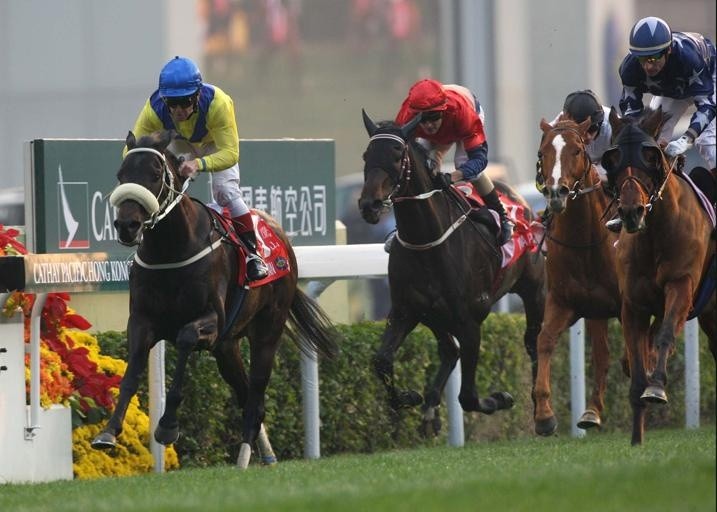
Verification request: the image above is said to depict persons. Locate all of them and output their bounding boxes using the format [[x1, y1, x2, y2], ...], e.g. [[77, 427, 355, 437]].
[[619, 15, 717, 179], [393, 78, 516, 247], [121, 54, 269, 282], [536, 88, 621, 203]]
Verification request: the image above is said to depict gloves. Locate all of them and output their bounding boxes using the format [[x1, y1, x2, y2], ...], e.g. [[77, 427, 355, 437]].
[[663, 135, 694, 160]]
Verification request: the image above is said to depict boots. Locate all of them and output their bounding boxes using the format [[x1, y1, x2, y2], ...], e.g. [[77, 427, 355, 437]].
[[240, 231, 269, 280], [480, 187, 517, 247]]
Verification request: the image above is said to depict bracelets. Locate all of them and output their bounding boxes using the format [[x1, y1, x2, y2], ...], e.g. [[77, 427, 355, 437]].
[[685, 131, 696, 144]]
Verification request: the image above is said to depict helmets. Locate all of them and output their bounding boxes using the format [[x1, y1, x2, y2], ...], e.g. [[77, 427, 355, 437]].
[[564, 89, 604, 127], [628, 17, 672, 56], [408, 80, 448, 114], [158, 56, 203, 97]]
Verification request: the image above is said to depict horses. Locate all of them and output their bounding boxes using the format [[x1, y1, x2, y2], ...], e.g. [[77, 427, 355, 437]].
[[533, 117, 676, 436], [600, 104, 717, 447], [90, 130, 339, 469], [358, 107, 551, 437]]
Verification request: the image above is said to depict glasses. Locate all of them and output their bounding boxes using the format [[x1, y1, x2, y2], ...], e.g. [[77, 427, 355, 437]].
[[160, 89, 200, 109], [416, 113, 442, 122], [635, 49, 666, 66]]
